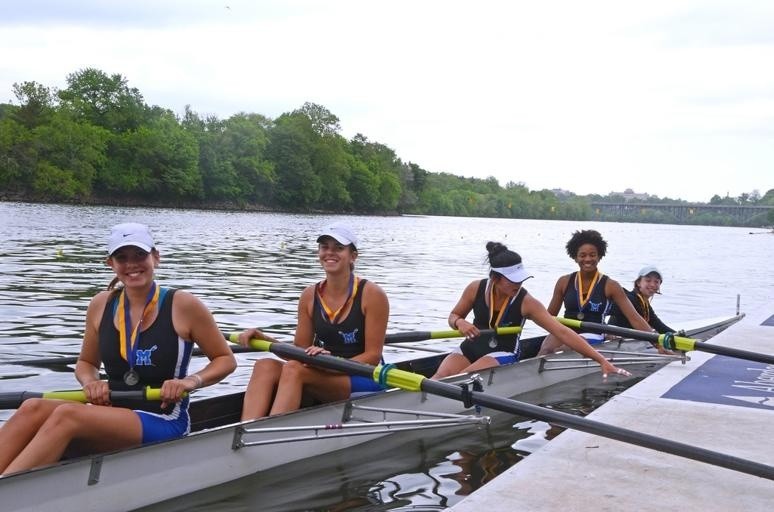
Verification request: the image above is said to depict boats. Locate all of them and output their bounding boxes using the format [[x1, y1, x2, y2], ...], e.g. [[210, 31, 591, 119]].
[[1, 294, 746, 510]]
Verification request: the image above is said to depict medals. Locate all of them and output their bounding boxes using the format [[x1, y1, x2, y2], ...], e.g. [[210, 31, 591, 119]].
[[575, 310, 585, 320], [123, 369, 141, 386], [488, 332, 503, 350]]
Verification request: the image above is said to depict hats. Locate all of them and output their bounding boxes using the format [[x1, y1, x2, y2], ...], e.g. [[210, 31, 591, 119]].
[[639, 267, 663, 283], [107, 223, 155, 256], [491, 262, 534, 283], [316, 226, 359, 251]]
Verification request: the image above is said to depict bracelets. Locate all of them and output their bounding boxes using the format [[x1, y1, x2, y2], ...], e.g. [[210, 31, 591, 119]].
[[186, 371, 209, 386]]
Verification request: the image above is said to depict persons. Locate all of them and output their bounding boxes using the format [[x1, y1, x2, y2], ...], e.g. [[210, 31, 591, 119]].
[[237, 224, 390, 426], [607, 264, 684, 340], [0, 222, 238, 482], [536, 227, 690, 365], [419, 242, 632, 385]]
